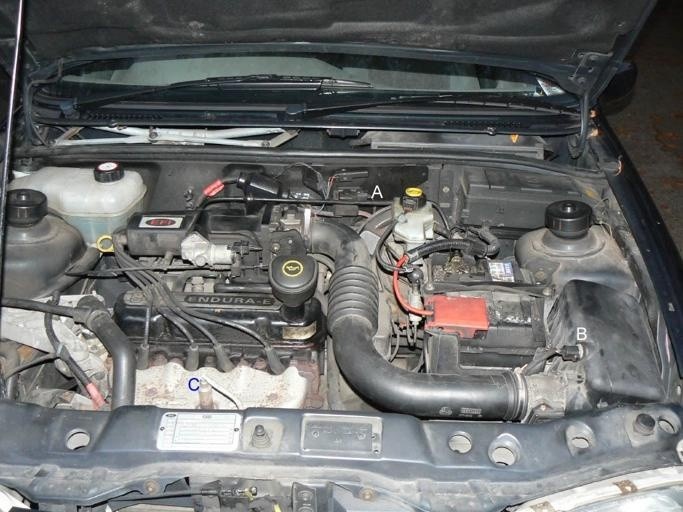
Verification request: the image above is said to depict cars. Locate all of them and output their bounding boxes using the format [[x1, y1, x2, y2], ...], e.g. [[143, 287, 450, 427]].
[[0, 0, 683, 512]]
